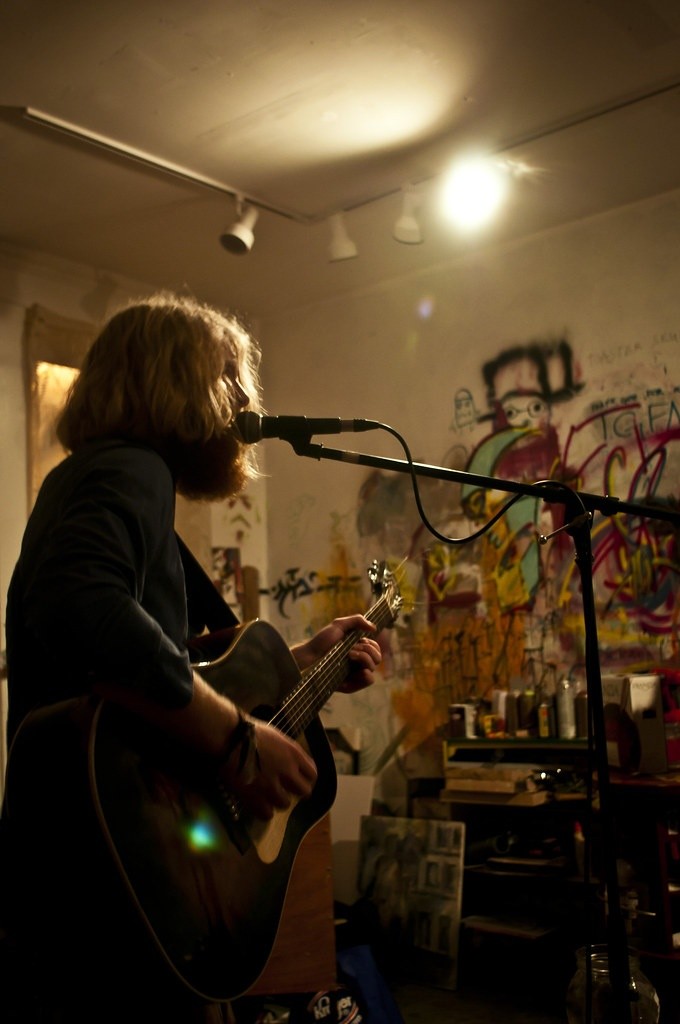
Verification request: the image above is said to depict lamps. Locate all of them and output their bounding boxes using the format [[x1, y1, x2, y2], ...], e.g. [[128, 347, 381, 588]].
[[392, 186, 422, 243], [329, 212, 359, 263], [221, 197, 260, 256]]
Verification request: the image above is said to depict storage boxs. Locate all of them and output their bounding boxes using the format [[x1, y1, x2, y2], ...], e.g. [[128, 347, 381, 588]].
[[442, 762, 547, 807], [447, 703, 477, 743]]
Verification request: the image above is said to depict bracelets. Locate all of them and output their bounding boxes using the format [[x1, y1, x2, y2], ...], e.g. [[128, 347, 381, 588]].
[[215, 707, 261, 778]]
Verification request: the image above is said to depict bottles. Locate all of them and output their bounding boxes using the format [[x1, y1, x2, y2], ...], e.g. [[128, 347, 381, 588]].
[[555, 680, 576, 739]]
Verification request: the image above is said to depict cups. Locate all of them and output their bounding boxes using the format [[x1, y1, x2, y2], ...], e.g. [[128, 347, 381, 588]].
[[448, 703, 475, 737]]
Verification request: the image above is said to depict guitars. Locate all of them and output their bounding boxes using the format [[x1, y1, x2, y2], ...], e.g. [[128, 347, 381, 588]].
[[0, 556, 402, 1014]]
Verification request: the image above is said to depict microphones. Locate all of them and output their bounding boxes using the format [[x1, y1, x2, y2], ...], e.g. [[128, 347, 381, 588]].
[[231, 410, 382, 445]]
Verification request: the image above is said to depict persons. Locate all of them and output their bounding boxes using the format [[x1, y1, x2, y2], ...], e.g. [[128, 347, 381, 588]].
[[0, 303, 383, 1024]]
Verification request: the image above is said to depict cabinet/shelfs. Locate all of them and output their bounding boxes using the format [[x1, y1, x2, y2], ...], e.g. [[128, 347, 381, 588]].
[[596, 772, 680, 968], [446, 736, 616, 998]]
